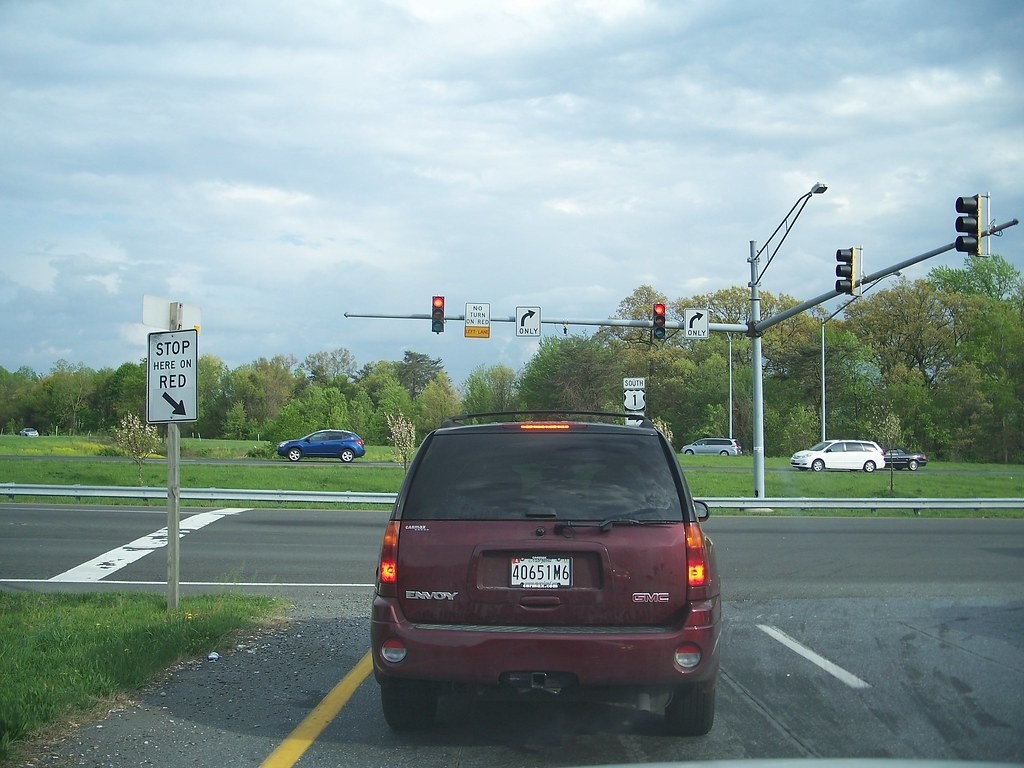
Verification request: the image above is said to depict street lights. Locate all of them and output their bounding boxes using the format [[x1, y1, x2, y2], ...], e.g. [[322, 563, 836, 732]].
[[747, 181, 829, 497], [821, 270, 902, 443]]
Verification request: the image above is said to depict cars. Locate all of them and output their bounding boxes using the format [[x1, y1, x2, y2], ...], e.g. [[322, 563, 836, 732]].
[[680, 438, 742, 458], [277, 429, 366, 463], [19, 427, 40, 439], [882, 448, 929, 471]]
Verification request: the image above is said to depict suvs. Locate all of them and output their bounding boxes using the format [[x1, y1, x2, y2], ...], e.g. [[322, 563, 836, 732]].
[[369, 409, 721, 735], [789, 439, 887, 472]]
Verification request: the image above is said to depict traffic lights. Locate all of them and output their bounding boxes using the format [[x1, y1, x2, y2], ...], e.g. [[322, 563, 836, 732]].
[[431, 296, 445, 333], [835, 247, 856, 295], [955, 193, 983, 258], [652, 301, 668, 341]]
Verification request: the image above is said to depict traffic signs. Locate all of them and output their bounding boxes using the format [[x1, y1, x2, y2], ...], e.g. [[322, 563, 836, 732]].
[[147, 329, 199, 425]]
